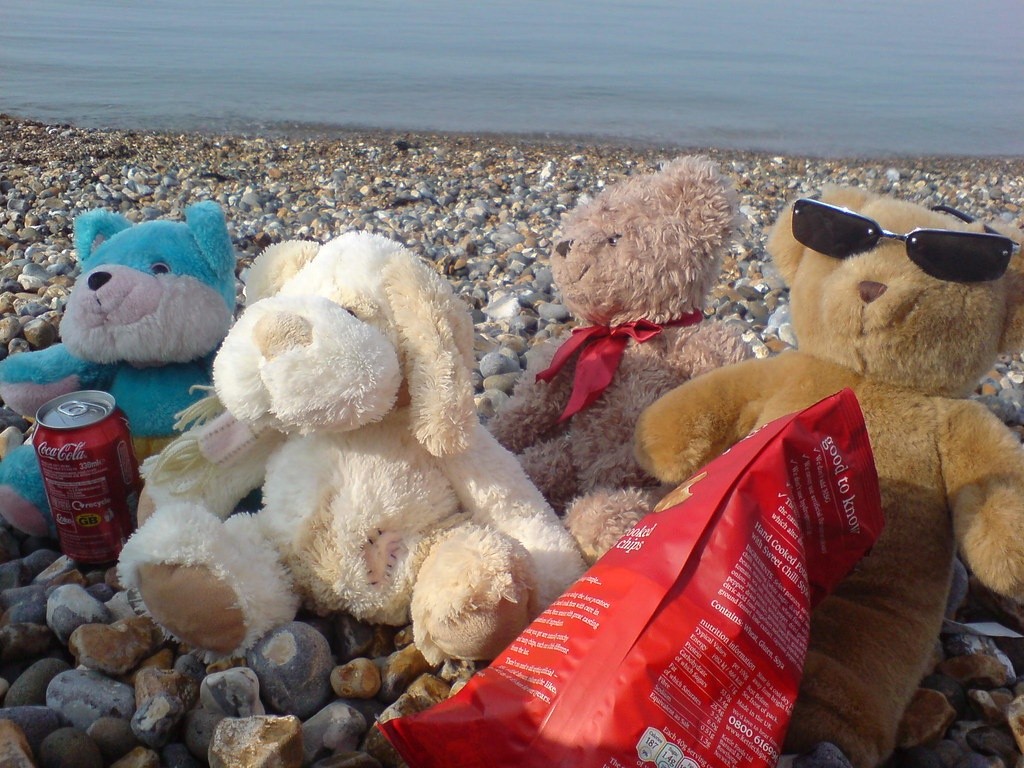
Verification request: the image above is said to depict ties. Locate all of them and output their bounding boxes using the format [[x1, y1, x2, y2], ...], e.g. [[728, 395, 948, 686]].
[[526, 314, 713, 416]]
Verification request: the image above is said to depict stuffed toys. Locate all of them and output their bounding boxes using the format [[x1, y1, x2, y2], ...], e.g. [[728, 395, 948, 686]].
[[0, 200, 261, 537], [484, 155, 755, 569], [117, 232, 590, 678], [634, 188, 1024, 768]]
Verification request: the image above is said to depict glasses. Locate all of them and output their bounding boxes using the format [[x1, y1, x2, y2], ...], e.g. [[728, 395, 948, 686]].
[[792, 191, 1018, 285]]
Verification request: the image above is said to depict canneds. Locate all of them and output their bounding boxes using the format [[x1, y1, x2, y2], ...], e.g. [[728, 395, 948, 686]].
[[35, 389, 143, 567]]
